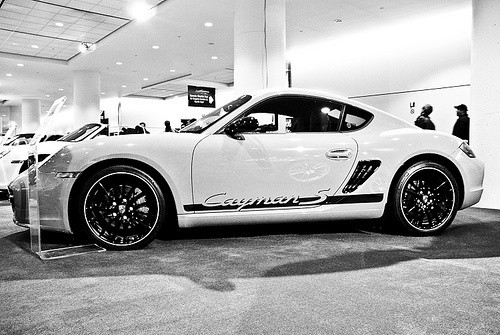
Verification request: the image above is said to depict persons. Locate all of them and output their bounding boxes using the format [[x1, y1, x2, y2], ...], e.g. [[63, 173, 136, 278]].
[[137, 118, 197, 134], [451, 103, 470, 145], [414, 104, 436, 130]]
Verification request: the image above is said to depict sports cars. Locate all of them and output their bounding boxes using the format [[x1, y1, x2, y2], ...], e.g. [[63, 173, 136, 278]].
[[0, 121, 109, 191], [6, 88, 486, 251]]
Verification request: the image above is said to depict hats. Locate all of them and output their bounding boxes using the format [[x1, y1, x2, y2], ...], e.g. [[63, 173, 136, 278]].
[[454, 104, 467, 112]]
